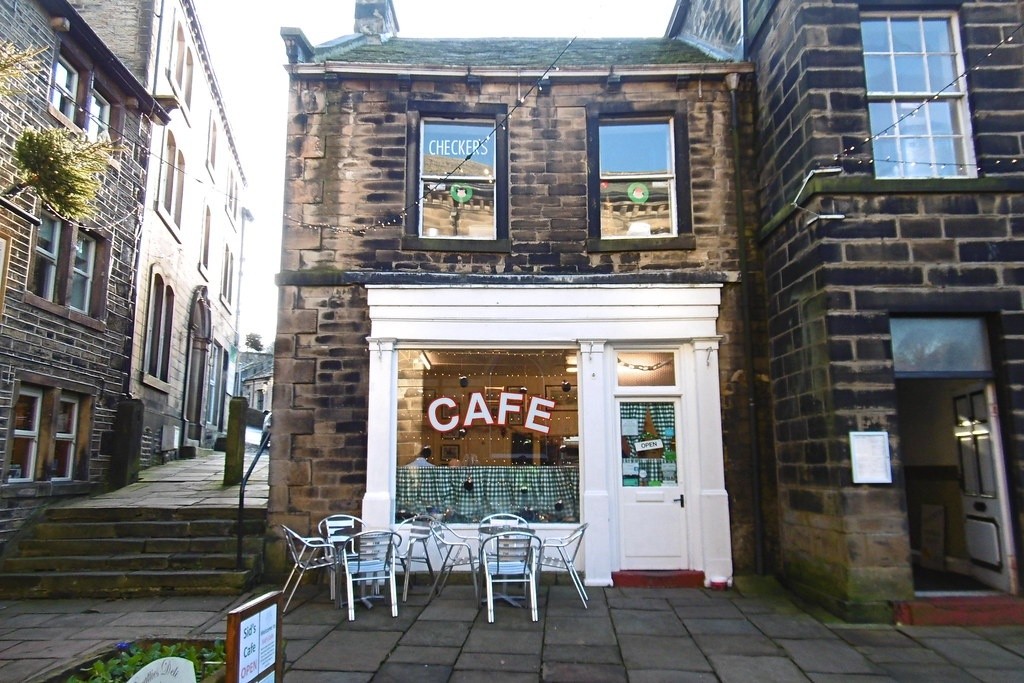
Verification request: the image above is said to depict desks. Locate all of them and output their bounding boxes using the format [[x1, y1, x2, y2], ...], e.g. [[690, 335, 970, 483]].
[[478, 526, 536, 608], [332, 528, 386, 601]]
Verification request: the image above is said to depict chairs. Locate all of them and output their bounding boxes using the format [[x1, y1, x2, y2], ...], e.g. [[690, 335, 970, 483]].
[[535, 522, 590, 609], [480, 532, 543, 623], [318, 514, 370, 608], [344, 529, 403, 621], [280, 525, 340, 613], [477, 512, 532, 606], [428, 520, 480, 604], [388, 515, 440, 604]]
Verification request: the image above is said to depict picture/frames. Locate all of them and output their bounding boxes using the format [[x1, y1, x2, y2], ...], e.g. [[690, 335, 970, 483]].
[[441, 402, 461, 420], [508, 407, 524, 426], [441, 443, 460, 461], [424, 388, 436, 399], [483, 385, 504, 402], [441, 427, 461, 439], [507, 386, 525, 405], [544, 384, 578, 412]]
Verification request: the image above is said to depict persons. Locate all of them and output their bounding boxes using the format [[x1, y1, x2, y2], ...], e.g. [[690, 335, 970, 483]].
[[447, 450, 461, 466], [260, 410, 272, 448], [408, 448, 436, 466]]
